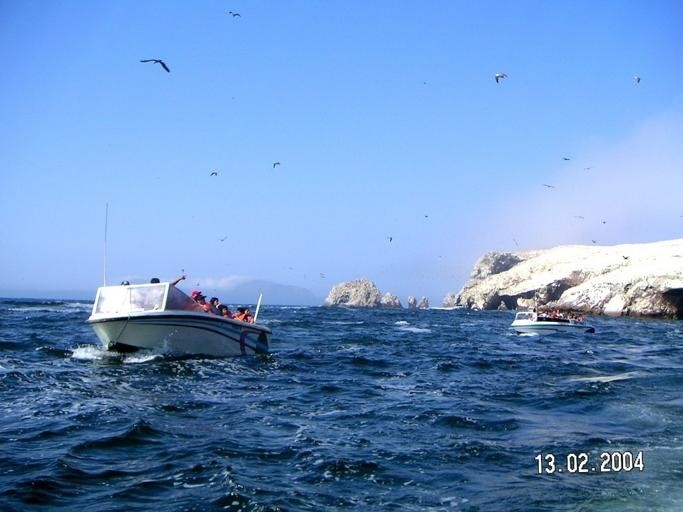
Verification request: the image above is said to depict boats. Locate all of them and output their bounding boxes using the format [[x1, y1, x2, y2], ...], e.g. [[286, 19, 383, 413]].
[[511, 311, 594, 336], [85, 283, 272, 356]]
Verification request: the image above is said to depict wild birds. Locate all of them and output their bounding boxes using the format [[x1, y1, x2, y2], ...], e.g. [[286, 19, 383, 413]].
[[227, 10, 239, 17], [138, 58, 169, 73], [272, 161, 280, 168], [208, 170, 217, 178], [495, 73, 507, 84], [634, 76, 641, 85]]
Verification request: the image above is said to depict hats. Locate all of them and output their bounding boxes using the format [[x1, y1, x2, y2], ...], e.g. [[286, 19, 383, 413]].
[[196, 296, 207, 301], [192, 290, 201, 296]]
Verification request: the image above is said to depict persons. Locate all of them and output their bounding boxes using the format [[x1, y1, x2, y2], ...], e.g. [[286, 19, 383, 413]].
[[121, 276, 254, 323], [540, 303, 584, 321]]
